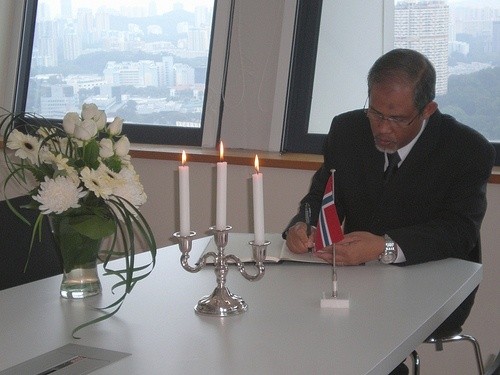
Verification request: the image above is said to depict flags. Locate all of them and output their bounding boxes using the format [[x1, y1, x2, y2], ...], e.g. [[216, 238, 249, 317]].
[[315, 176, 344, 251]]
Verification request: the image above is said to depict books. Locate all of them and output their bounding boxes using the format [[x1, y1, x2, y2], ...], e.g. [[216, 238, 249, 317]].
[[195, 233, 329, 265]]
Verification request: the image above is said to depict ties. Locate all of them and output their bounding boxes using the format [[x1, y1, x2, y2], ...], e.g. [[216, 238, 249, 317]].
[[383, 150, 401, 191]]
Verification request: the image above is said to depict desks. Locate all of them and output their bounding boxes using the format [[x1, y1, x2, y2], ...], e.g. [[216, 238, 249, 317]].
[[0, 233, 483, 375]]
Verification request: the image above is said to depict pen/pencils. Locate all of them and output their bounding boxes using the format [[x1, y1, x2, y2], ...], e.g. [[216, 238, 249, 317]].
[[305, 203, 312, 255], [331, 242, 338, 297]]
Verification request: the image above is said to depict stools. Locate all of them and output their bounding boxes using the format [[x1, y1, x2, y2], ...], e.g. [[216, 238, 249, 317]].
[[409, 326, 486, 375]]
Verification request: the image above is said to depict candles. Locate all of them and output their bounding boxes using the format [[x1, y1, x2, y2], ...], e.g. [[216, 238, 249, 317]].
[[216, 140, 227, 229], [251, 153, 264, 243], [178, 149, 191, 236]]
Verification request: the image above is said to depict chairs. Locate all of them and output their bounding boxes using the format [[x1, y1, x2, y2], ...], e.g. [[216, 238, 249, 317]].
[[0, 194, 63, 290]]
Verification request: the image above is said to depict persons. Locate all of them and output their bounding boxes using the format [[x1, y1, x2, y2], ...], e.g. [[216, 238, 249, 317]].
[[282, 48, 494, 375]]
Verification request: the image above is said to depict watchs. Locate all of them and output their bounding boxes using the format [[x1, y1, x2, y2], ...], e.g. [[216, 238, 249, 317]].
[[377, 233, 396, 265]]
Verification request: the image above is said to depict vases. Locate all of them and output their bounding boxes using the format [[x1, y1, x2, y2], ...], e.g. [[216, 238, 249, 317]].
[[45, 212, 101, 299]]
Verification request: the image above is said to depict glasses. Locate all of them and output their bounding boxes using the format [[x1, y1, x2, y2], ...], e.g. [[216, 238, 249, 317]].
[[363, 96, 426, 126]]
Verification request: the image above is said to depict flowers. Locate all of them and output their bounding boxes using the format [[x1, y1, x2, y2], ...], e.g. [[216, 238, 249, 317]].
[[0, 103, 156, 340]]
